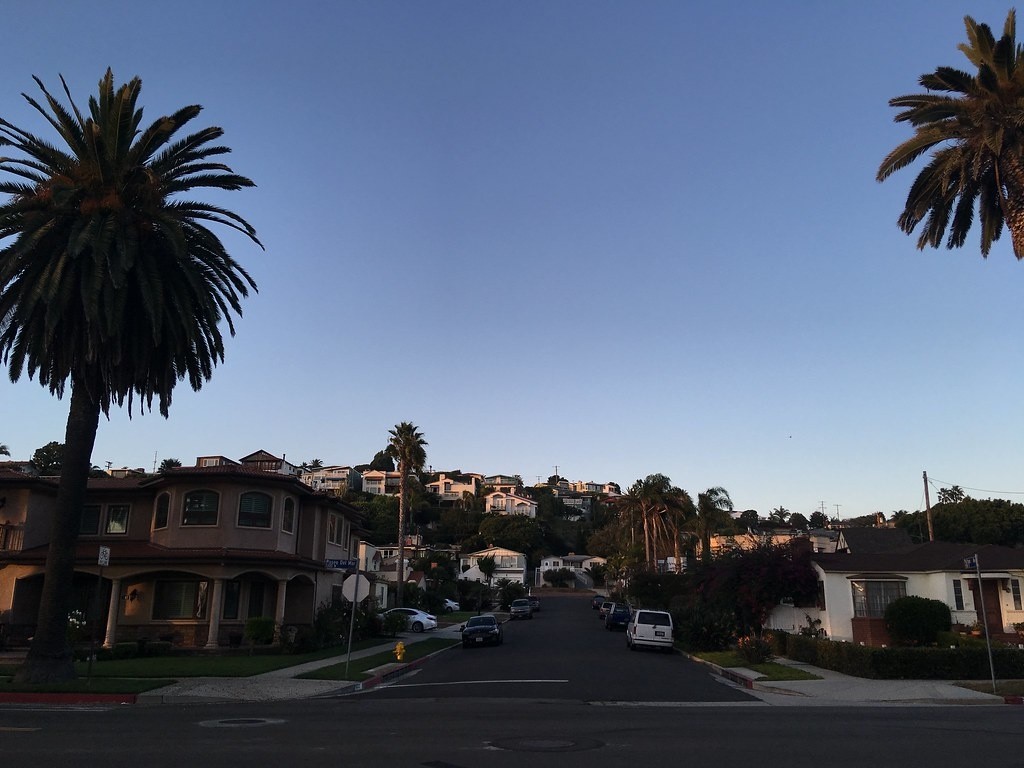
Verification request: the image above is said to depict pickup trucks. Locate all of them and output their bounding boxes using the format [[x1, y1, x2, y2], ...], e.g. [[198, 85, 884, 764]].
[[605, 603, 631, 631]]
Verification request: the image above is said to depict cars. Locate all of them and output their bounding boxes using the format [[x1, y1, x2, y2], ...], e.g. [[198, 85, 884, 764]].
[[600, 602, 615, 619], [508, 599, 532, 620], [442, 599, 460, 612], [592, 595, 606, 610], [376, 607, 437, 633], [530, 601, 541, 611], [460, 615, 504, 646]]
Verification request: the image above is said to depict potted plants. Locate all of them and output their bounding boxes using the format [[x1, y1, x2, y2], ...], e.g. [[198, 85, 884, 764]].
[[971, 620, 985, 638], [1013, 622, 1024, 639], [952, 615, 965, 632]]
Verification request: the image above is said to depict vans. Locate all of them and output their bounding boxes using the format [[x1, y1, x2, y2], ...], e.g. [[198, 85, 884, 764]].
[[626, 609, 674, 652]]
